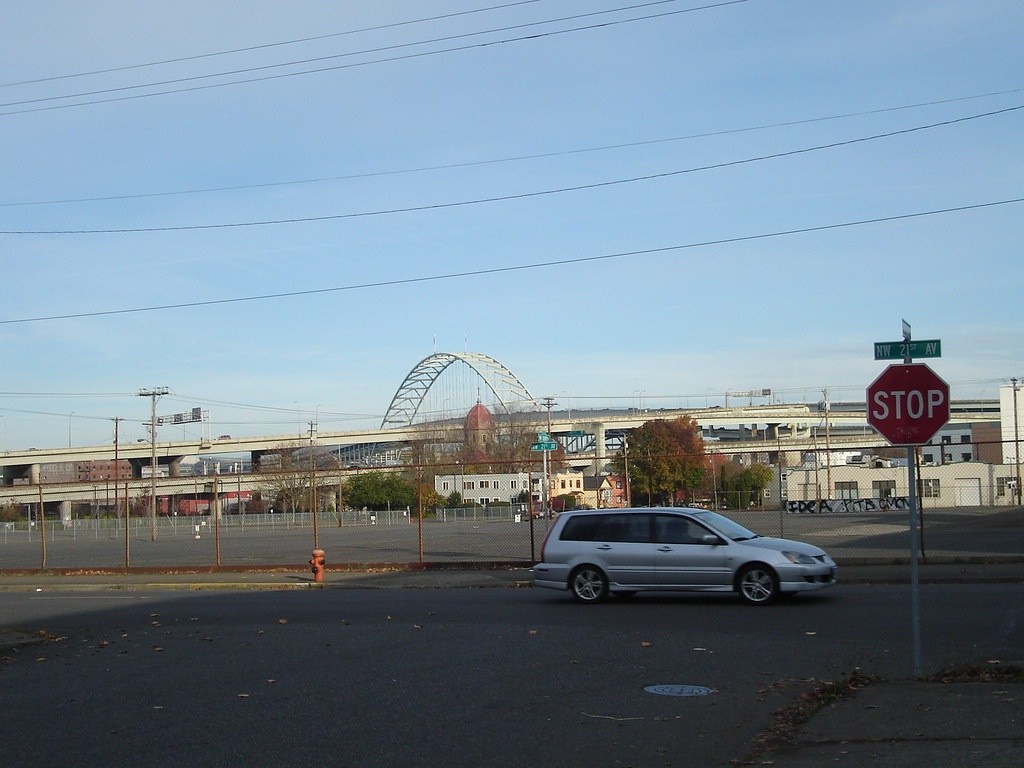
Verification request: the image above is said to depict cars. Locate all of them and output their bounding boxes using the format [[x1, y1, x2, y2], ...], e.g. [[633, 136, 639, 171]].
[[532, 505, 841, 606]]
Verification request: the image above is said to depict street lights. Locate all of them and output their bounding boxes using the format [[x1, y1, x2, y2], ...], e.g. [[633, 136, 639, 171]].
[[725, 386, 734, 408], [632, 390, 640, 413], [68, 411, 75, 449], [639, 389, 647, 411], [135, 437, 157, 542], [706, 387, 714, 408], [316, 403, 322, 433], [443, 398, 450, 419]]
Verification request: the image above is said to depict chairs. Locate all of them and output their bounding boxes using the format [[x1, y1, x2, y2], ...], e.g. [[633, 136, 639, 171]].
[[665, 519, 686, 545]]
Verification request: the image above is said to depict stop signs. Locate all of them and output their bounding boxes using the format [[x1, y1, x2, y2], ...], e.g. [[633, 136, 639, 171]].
[[864, 364, 952, 446]]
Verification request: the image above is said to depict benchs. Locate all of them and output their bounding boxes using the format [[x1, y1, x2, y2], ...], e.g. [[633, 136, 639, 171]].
[[569, 522, 636, 543]]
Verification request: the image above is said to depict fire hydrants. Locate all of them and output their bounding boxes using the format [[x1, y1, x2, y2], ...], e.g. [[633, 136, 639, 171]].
[[308, 549, 325, 583]]
